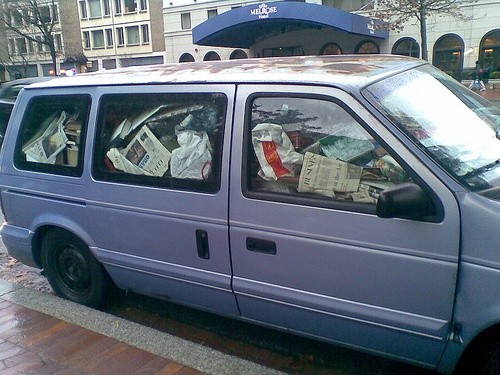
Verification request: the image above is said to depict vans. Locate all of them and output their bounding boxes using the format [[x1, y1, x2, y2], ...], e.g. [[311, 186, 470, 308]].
[[0, 54, 500, 374]]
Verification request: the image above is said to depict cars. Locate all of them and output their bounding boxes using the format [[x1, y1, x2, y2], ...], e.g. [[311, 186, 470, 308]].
[[0, 77, 59, 146]]
